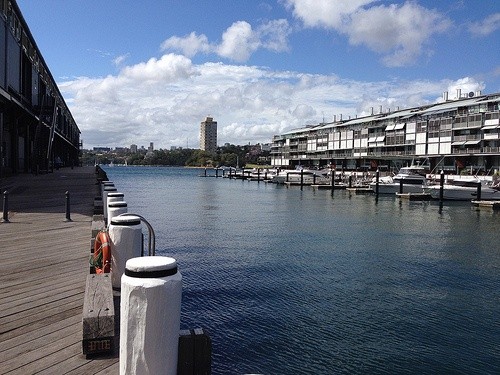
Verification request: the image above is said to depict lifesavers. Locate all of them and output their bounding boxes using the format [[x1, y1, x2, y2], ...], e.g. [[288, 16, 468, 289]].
[[93, 230, 111, 274]]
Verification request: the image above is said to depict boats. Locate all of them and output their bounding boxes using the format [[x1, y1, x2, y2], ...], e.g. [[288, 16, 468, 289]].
[[215, 153, 500, 200]]
